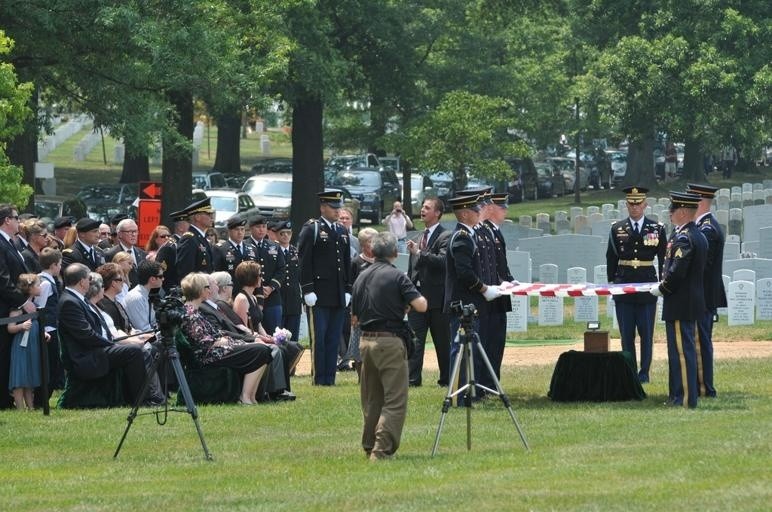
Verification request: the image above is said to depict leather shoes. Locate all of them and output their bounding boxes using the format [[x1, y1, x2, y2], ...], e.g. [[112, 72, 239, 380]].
[[276, 392, 289, 400], [637, 369, 649, 382], [283, 388, 297, 401]]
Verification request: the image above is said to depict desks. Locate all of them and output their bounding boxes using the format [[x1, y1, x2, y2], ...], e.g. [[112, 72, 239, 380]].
[[545, 349, 646, 401]]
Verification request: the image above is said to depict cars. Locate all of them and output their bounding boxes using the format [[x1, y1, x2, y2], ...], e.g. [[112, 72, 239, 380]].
[[205, 187, 259, 231], [74, 183, 135, 224], [193, 170, 230, 195], [33, 193, 89, 221]]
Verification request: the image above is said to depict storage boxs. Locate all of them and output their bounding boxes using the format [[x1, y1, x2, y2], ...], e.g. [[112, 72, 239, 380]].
[[584, 331, 610, 352]]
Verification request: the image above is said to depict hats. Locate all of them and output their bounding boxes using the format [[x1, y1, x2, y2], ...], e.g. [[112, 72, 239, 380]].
[[52, 215, 77, 227], [76, 216, 103, 233], [685, 183, 719, 200], [667, 192, 703, 209], [111, 213, 128, 225], [316, 190, 345, 210], [19, 212, 38, 222], [621, 185, 647, 203], [448, 185, 509, 210], [225, 215, 294, 232], [169, 197, 216, 221]]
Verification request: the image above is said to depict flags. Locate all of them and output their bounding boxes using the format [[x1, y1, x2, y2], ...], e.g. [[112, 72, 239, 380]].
[[499, 281, 661, 297]]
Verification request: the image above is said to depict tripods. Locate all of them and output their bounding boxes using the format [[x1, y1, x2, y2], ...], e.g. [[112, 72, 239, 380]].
[[114, 338, 214, 463], [432, 323, 533, 462]]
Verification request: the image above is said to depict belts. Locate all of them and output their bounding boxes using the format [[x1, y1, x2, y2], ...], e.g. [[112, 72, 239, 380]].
[[361, 330, 398, 337]]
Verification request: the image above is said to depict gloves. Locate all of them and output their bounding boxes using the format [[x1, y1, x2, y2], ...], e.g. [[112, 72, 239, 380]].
[[650, 281, 660, 295], [486, 281, 516, 300], [304, 292, 317, 306]]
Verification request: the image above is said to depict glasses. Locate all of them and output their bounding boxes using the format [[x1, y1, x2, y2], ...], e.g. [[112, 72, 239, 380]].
[[150, 274, 163, 279], [2, 215, 18, 220], [39, 233, 47, 238]]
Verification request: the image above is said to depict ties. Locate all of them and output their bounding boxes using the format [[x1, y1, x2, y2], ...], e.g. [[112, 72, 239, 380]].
[[127, 249, 131, 255], [257, 242, 261, 248], [634, 222, 639, 233], [421, 229, 430, 250], [89, 248, 93, 258], [284, 248, 288, 255], [330, 224, 335, 233], [236, 245, 242, 254]]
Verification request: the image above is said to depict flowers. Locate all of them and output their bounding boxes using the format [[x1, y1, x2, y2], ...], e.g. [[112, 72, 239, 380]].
[[270, 325, 293, 350]]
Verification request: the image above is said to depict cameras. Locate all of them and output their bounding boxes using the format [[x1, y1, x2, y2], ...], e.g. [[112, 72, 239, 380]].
[[452, 301, 479, 323]]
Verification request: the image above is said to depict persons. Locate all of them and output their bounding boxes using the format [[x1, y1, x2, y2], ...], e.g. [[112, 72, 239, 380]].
[[650, 189, 709, 409], [606, 185, 668, 382], [684, 184, 728, 397]]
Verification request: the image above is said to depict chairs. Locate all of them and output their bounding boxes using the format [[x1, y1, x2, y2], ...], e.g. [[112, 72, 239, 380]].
[[55, 320, 263, 410]]
